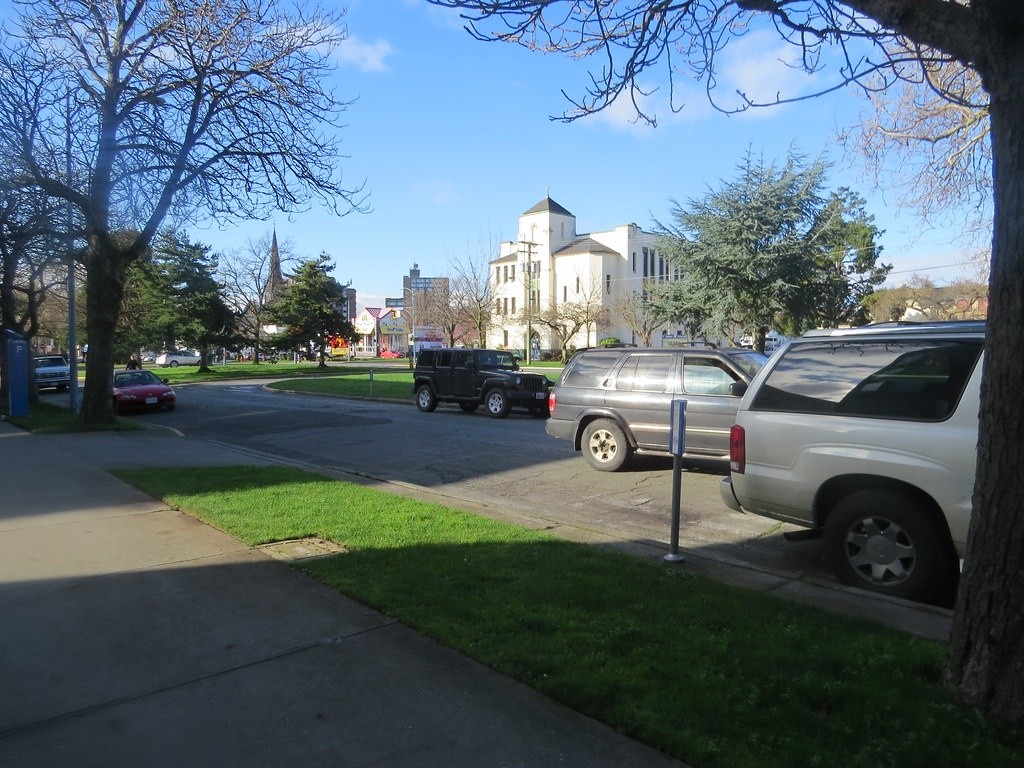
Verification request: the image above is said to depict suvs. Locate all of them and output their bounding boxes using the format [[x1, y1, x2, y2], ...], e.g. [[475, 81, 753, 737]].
[[718, 316, 988, 604], [410, 346, 552, 420], [32, 355, 71, 390], [542, 341, 775, 472]]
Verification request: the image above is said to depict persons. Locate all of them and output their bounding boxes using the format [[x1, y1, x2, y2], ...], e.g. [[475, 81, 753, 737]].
[[126, 356, 140, 370]]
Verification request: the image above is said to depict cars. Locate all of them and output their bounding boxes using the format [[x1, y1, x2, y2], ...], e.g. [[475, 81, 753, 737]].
[[112, 368, 176, 414], [139, 353, 156, 363], [242, 352, 279, 365], [157, 348, 202, 368], [381, 350, 404, 359]]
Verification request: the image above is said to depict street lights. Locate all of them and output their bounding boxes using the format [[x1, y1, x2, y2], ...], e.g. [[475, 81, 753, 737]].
[[62, 85, 167, 415], [399, 286, 417, 368]]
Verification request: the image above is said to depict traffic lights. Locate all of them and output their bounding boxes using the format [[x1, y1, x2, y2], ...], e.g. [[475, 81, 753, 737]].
[[391, 308, 397, 319]]
